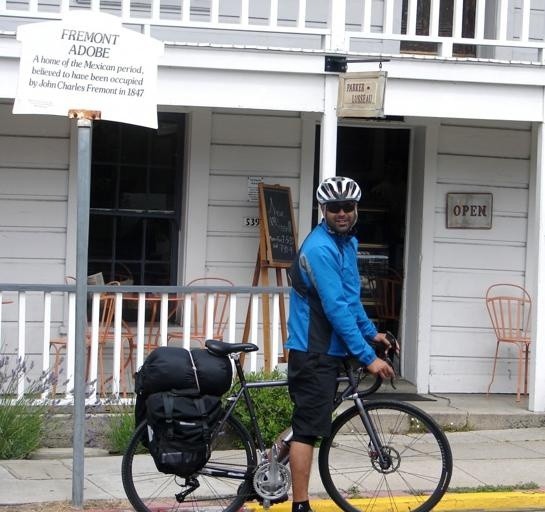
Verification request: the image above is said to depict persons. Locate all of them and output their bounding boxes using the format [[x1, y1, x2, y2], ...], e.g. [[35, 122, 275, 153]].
[[258, 175, 402, 512]]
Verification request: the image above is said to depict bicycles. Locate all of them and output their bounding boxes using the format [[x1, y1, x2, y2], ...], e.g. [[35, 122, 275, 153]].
[[122, 335, 454, 512]]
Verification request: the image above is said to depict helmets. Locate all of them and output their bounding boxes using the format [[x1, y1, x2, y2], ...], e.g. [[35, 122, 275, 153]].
[[315, 175, 363, 205]]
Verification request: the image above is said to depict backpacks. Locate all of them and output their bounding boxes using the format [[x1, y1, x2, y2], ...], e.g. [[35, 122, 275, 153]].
[[145, 391, 223, 481]]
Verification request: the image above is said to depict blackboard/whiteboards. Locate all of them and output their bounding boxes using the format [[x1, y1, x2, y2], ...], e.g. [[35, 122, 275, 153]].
[[258, 184, 299, 268]]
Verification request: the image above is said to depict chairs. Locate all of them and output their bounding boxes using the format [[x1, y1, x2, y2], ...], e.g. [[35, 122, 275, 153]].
[[485, 283, 532, 406], [51, 276, 236, 403], [363, 268, 402, 372]]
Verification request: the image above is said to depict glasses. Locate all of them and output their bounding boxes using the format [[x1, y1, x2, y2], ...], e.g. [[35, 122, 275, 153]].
[[327, 203, 354, 214]]
